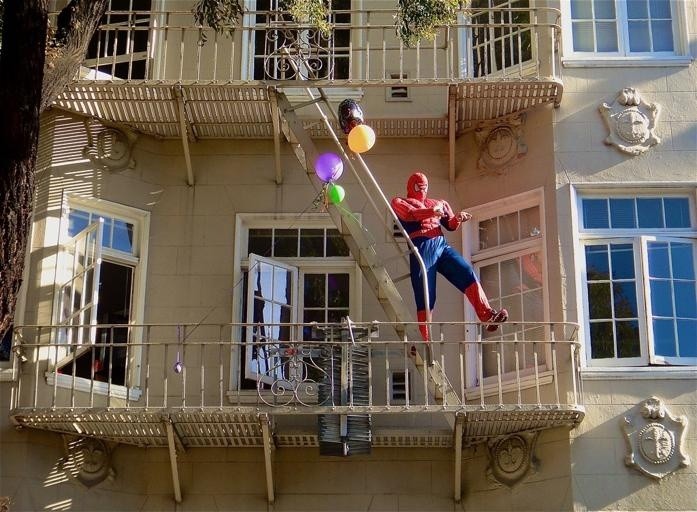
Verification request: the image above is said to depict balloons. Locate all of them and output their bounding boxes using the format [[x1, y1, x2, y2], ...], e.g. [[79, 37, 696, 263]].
[[314, 153, 345, 182], [348, 125, 376, 153], [328, 185, 345, 204], [338, 99, 365, 134]]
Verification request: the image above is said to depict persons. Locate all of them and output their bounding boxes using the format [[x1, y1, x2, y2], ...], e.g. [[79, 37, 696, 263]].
[[391, 173, 509, 341]]
